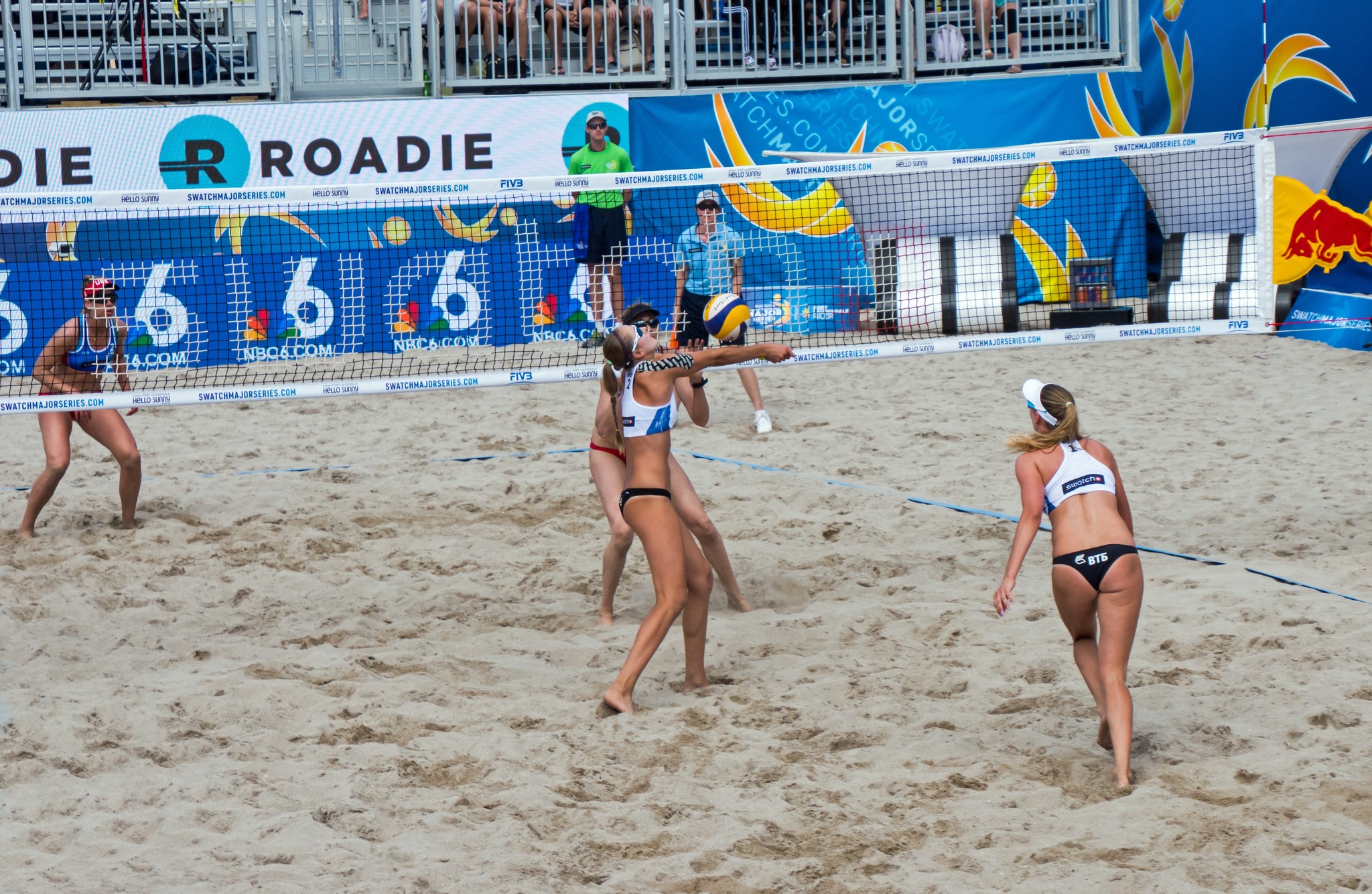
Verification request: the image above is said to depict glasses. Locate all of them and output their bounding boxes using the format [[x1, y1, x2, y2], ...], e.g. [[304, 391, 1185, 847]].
[[625, 325, 643, 367], [632, 318, 660, 328], [89, 293, 118, 304], [588, 123, 606, 130], [696, 204, 719, 211]]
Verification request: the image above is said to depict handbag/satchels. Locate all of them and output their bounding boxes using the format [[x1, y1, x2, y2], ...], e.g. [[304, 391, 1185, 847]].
[[150, 41, 217, 85], [483, 52, 530, 95]]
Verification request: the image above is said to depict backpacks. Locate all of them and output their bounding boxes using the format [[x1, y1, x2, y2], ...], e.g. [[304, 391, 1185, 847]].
[[930, 24, 968, 76], [613, 25, 649, 71]]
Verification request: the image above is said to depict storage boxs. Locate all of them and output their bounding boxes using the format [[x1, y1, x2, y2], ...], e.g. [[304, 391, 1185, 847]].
[[1069, 257, 1114, 311]]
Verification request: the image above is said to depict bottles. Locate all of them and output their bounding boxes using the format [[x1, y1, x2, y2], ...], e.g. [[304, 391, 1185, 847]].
[[423, 68, 431, 96]]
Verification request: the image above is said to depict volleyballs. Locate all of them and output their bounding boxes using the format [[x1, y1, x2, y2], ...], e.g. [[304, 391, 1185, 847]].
[[702, 292, 751, 341]]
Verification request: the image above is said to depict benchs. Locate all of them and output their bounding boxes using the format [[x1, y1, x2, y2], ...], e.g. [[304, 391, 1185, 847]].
[[0, 0, 259, 103], [348, 0, 1122, 95]]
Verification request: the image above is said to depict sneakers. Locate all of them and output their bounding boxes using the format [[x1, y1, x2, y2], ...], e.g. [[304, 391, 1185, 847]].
[[741, 56, 760, 69], [765, 56, 779, 69], [753, 412, 773, 434]]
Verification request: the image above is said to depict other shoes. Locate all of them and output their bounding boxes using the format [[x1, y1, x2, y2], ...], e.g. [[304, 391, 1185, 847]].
[[811, 3, 831, 22], [456, 49, 473, 66], [793, 53, 803, 67], [607, 61, 623, 73], [525, 60, 535, 76], [822, 24, 836, 40], [648, 60, 655, 72], [582, 330, 608, 348], [834, 50, 851, 66], [423, 46, 445, 69]]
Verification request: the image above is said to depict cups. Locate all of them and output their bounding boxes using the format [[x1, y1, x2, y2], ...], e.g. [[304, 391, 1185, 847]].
[[440, 76, 453, 96], [474, 59, 486, 75]]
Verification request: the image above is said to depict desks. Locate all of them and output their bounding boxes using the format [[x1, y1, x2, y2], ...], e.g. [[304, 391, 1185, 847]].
[[1049, 305, 1134, 330]]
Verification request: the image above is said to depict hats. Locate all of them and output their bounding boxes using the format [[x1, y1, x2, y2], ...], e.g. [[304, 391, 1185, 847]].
[[82, 279, 121, 298], [696, 190, 720, 205], [623, 305, 660, 325], [1022, 378, 1064, 425], [586, 110, 607, 124]]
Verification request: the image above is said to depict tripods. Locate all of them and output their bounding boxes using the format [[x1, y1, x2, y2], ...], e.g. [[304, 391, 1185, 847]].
[[80, 0, 245, 91]]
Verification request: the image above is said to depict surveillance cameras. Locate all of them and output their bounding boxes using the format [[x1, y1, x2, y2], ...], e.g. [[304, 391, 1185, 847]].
[[48, 242, 73, 257]]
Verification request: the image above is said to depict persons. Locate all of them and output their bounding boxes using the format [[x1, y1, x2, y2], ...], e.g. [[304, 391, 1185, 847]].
[[20, 275, 141, 539], [421, 0, 536, 76], [877, 0, 929, 60], [671, 189, 775, 434], [680, 0, 861, 67], [534, 0, 606, 73], [585, 0, 655, 74], [589, 303, 795, 714], [358, 0, 368, 19], [993, 380, 1144, 791], [568, 110, 635, 349], [972, 0, 1022, 73]]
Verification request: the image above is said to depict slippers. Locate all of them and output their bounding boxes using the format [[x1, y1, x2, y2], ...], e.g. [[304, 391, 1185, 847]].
[[550, 66, 565, 74], [1006, 65, 1022, 73], [583, 65, 606, 73], [980, 49, 994, 57]]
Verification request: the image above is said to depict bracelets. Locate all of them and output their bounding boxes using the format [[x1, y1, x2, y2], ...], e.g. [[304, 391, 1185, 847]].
[[689, 376, 708, 388]]
